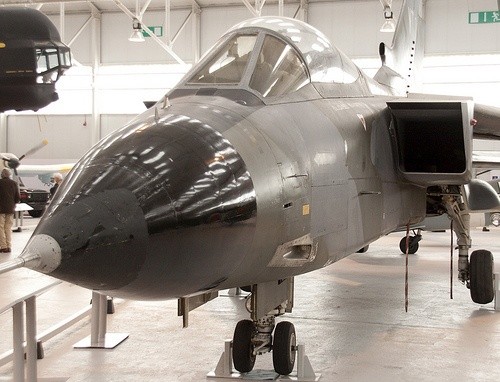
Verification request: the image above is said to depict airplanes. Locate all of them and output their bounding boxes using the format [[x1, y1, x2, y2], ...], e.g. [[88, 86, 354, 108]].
[[2, 2, 500, 379]]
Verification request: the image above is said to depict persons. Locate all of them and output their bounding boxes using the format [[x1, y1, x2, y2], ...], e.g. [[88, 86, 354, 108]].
[[50, 173, 63, 195], [0, 169, 21, 253]]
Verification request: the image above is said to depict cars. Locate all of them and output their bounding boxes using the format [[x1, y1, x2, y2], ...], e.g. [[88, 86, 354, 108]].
[[8, 175, 50, 217]]
